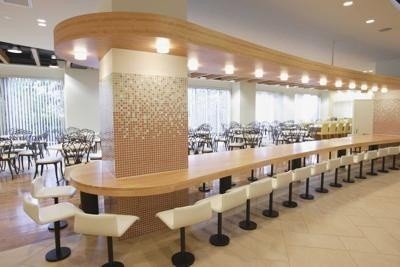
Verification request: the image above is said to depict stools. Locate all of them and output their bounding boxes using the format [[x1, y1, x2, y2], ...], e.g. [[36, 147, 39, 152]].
[[21, 197, 76, 263], [342, 151, 365, 184], [272, 166, 310, 209], [376, 147, 390, 174], [330, 155, 353, 188], [195, 187, 246, 247], [301, 162, 327, 201], [364, 149, 378, 176], [225, 178, 274, 231], [73, 208, 140, 266], [31, 175, 77, 233], [389, 145, 400, 171], [155, 200, 213, 266], [250, 170, 293, 219]]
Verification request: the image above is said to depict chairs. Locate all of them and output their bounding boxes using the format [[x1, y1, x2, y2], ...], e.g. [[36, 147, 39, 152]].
[[187, 117, 353, 157], [1, 126, 109, 184]]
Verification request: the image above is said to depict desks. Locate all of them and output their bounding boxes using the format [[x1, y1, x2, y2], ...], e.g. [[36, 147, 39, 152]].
[[62, 133, 400, 239]]
